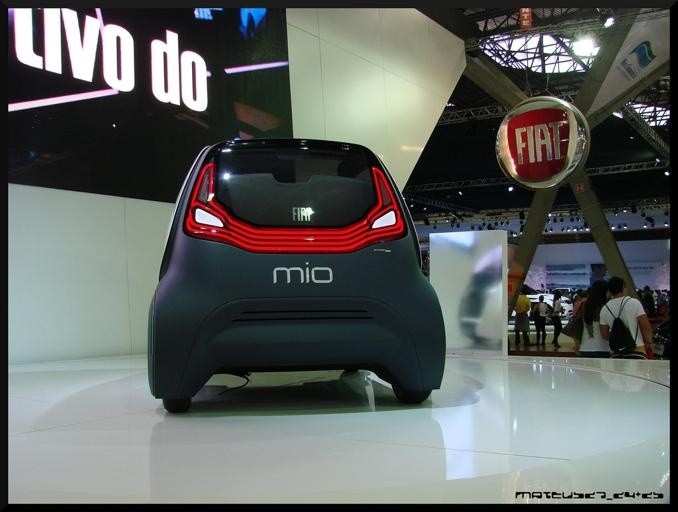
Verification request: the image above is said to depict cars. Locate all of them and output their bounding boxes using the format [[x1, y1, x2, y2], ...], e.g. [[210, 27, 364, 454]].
[[142, 137, 448, 414], [511, 293, 574, 325]]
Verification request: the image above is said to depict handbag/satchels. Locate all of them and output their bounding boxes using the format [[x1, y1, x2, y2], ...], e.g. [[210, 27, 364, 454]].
[[533, 303, 539, 320], [563, 301, 583, 340]]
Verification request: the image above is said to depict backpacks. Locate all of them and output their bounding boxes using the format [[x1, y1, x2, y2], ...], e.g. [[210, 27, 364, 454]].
[[604, 295, 638, 356]]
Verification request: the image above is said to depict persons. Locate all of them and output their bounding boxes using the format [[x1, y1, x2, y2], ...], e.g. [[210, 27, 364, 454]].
[[514, 275, 670, 361]]
